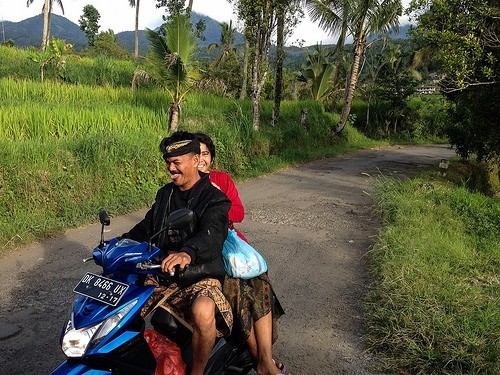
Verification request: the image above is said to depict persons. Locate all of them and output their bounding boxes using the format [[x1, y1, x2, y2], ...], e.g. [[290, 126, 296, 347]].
[[91, 131, 234, 375], [186, 131, 284, 375]]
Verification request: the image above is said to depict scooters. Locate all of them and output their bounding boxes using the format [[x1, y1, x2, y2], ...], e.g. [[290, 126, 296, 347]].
[[42, 204, 262, 374]]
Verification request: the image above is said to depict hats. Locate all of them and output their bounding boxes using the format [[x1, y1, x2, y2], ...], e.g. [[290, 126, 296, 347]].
[[160, 132, 200, 158]]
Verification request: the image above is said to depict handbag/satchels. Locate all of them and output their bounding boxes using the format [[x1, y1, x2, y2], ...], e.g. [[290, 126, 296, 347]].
[[145, 327, 187, 375], [222, 228, 268, 280]]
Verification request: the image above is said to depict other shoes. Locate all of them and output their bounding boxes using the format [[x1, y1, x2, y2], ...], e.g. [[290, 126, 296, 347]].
[[271, 358, 285, 373]]
